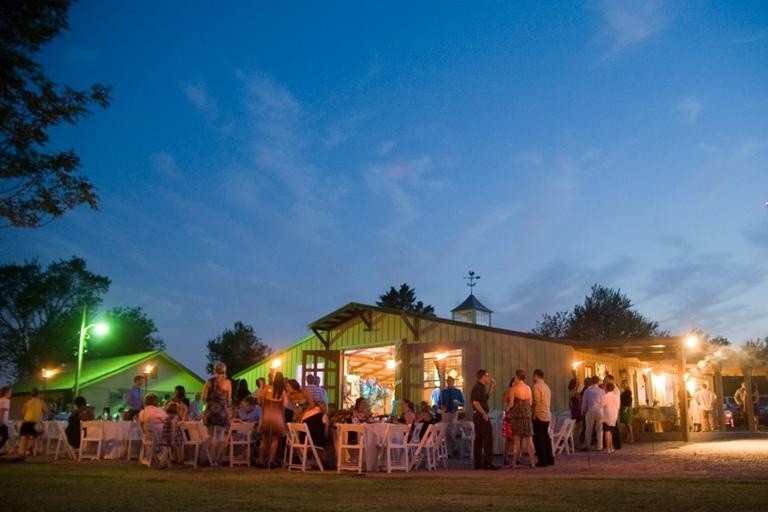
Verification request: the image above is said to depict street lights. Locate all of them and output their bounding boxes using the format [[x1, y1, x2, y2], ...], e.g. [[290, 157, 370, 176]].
[[40, 366, 51, 400], [269, 358, 282, 382], [143, 363, 152, 396], [73, 322, 110, 403]]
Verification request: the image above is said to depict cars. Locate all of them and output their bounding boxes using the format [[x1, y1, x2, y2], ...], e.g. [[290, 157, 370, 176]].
[[722, 393, 745, 429]]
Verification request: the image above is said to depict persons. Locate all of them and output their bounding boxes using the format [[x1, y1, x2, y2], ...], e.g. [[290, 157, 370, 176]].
[[199, 362, 231, 457], [122, 374, 204, 470], [470, 370, 497, 471], [65, 396, 93, 445], [500, 369, 556, 470], [733, 381, 746, 429], [347, 376, 465, 470], [743, 381, 758, 430], [677, 384, 693, 433], [20, 385, 47, 453], [568, 374, 633, 457], [695, 383, 717, 431], [229, 371, 330, 472], [0, 386, 13, 450]]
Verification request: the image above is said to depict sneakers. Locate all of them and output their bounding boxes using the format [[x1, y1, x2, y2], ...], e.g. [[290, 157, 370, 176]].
[[0, 447, 42, 457], [574, 445, 621, 453], [252, 458, 327, 468], [474, 459, 555, 471]]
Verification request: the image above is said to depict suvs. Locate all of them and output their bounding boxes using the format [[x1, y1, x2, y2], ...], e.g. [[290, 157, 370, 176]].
[[755, 392, 768, 428]]
